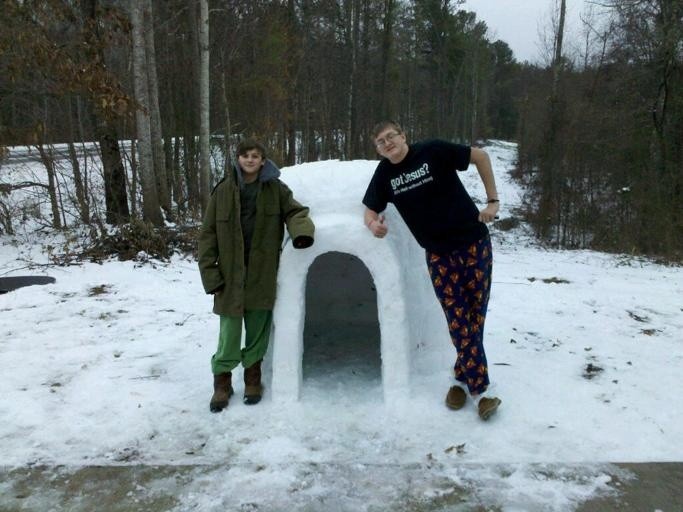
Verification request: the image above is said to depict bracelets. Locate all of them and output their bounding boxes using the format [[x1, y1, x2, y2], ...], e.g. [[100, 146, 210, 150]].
[[485, 197, 501, 205], [367, 219, 374, 229]]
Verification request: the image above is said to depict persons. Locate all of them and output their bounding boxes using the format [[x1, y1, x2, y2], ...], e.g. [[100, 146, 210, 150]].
[[195, 137, 317, 415], [362, 116, 503, 422]]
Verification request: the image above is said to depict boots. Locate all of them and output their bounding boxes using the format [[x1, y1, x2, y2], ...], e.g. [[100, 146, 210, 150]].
[[208, 371, 235, 414], [242, 359, 263, 406]]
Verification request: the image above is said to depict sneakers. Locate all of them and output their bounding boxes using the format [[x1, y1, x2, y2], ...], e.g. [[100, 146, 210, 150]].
[[475, 394, 502, 422], [443, 384, 467, 411]]
